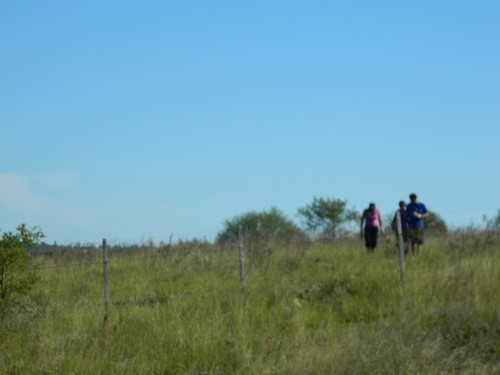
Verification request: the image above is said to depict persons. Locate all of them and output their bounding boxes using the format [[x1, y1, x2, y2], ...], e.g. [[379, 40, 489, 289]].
[[391, 201, 407, 253], [404, 193, 429, 257], [361, 202, 385, 254]]
